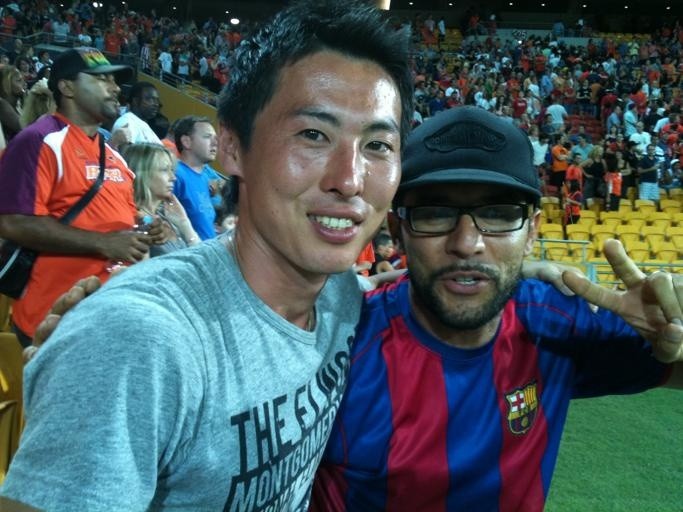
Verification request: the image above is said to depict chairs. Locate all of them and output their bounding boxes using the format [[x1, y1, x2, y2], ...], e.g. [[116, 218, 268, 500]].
[[529, 190, 683, 292]]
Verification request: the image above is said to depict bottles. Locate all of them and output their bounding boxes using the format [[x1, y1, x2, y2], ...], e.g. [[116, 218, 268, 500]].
[[104, 216, 153, 274]]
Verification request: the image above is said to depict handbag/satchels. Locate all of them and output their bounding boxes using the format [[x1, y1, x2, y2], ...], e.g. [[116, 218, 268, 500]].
[[0, 241, 39, 300]]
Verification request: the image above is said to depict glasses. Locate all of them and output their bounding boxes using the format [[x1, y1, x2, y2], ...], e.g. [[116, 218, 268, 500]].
[[394, 201, 531, 233]]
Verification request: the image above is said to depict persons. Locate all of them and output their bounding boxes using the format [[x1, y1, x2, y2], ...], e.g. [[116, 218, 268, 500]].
[[1, 64, 56, 150], [1, 0, 413, 511], [0, 46, 171, 349], [354, 234, 409, 291], [171, 115, 226, 240], [23, 107, 683, 511], [214, 176, 240, 236], [0, 0, 258, 95], [398, 12, 683, 223]]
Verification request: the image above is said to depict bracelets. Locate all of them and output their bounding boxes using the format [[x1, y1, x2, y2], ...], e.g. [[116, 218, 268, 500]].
[[186, 233, 199, 244]]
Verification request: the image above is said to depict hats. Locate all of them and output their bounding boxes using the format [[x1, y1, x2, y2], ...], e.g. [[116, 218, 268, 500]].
[[398, 105, 544, 198], [45, 44, 135, 85]]
